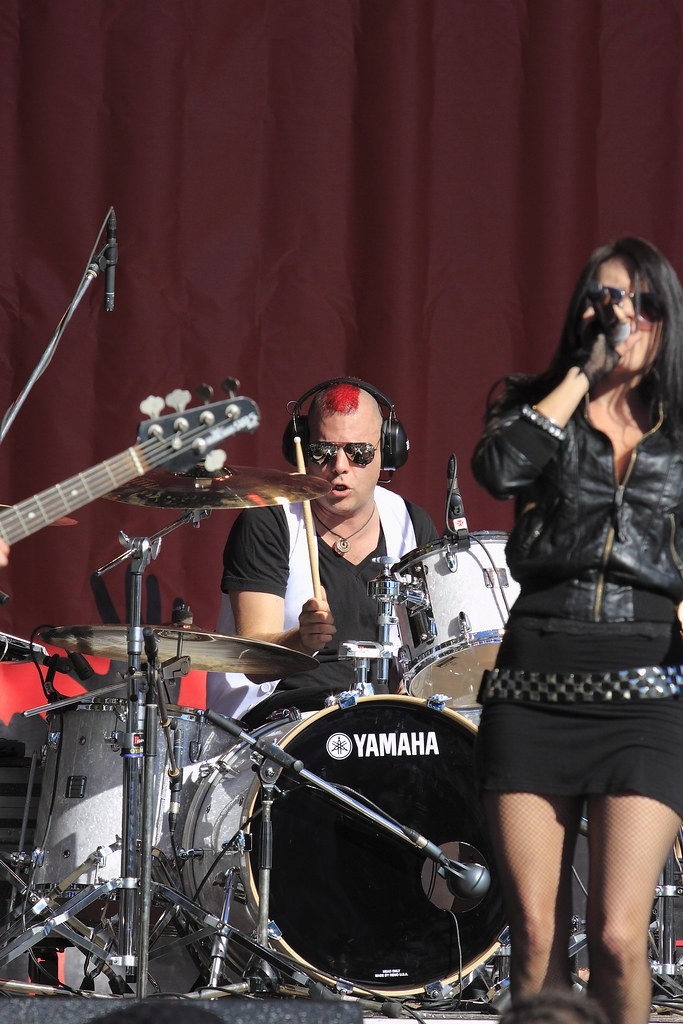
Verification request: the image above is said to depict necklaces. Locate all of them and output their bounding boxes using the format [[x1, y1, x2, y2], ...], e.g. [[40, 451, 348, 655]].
[[309, 501, 376, 556]]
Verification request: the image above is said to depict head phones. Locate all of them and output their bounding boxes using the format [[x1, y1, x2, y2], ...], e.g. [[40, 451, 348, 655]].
[[282, 378, 410, 471]]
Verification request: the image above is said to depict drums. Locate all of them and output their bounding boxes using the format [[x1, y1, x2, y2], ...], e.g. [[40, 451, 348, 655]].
[[23, 696, 235, 933], [177, 692, 516, 1000], [389, 529, 522, 714]]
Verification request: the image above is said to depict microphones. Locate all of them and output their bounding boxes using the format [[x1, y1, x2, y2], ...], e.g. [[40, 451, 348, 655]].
[[588, 281, 631, 347], [447, 857, 491, 900], [448, 458, 465, 518], [66, 649, 95, 680], [105, 209, 117, 311]]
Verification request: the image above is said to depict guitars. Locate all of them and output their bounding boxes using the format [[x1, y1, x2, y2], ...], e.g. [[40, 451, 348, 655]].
[[2, 376, 262, 546]]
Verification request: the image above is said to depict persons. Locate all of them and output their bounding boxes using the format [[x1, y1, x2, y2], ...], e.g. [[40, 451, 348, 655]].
[[470, 240, 683, 1023], [497, 989, 610, 1024], [207, 378, 441, 731]]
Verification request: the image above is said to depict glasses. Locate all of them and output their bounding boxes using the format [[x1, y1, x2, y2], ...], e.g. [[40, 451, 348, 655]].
[[582, 279, 671, 322], [302, 435, 383, 464]]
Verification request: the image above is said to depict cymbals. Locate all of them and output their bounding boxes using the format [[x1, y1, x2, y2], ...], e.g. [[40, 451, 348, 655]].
[[38, 620, 322, 676], [99, 462, 334, 511]]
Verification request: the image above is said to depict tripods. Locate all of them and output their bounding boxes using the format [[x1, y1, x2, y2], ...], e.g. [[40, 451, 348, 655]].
[[0, 505, 450, 1018]]
